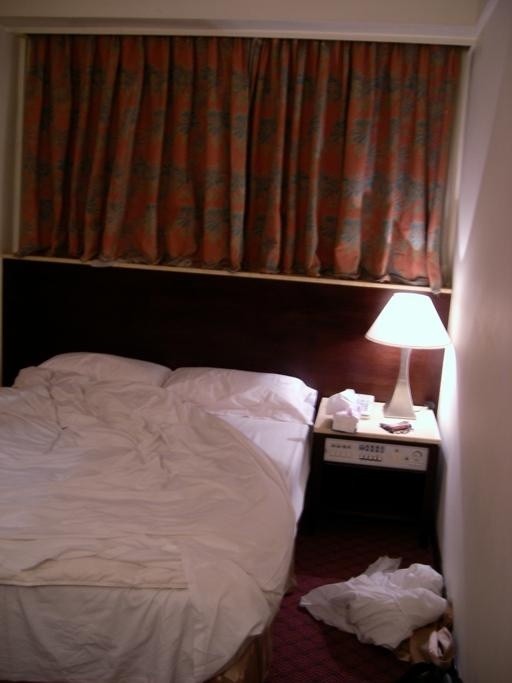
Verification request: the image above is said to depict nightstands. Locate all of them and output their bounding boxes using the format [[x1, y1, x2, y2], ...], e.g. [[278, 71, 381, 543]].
[[310, 396, 440, 580]]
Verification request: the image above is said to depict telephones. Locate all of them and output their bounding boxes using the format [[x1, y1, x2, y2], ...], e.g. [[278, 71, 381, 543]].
[[356, 393, 375, 420]]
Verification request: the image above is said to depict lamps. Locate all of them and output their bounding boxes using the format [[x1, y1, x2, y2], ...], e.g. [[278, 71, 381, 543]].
[[365, 290, 450, 420]]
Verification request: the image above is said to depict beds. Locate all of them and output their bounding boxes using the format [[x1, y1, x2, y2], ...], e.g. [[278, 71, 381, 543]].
[[0, 257, 451, 682]]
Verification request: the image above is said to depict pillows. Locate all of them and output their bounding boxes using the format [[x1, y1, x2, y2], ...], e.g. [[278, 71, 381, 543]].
[[38, 352, 170, 388], [162, 366, 313, 426]]
